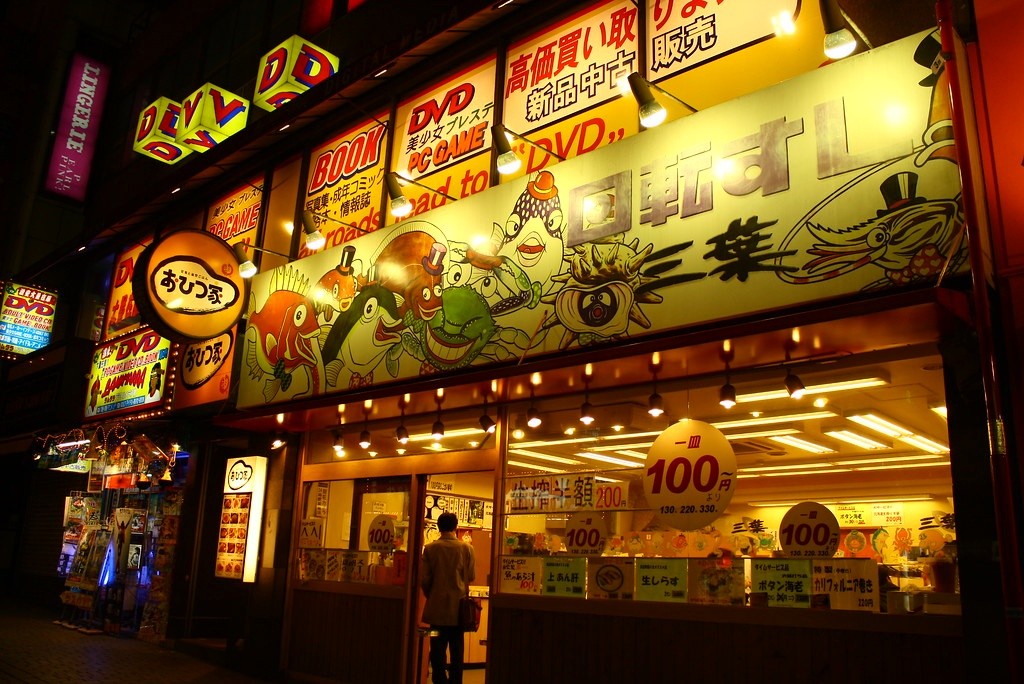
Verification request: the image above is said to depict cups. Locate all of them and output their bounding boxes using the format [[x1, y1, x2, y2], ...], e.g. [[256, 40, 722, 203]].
[[375, 565, 393, 583]]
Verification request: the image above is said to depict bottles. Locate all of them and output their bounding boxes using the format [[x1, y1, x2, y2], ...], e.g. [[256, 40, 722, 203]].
[[392, 550, 407, 586]]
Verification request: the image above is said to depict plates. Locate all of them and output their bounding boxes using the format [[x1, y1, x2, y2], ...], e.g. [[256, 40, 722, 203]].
[[596, 565, 624, 590]]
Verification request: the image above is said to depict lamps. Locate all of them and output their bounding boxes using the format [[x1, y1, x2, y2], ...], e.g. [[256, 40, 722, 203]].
[[394, 421, 405, 440], [479, 414, 497, 434], [299, 211, 367, 249], [627, 72, 698, 128], [232, 241, 294, 280], [818, 1, 873, 57], [508, 373, 947, 483], [491, 122, 564, 175], [360, 429, 373, 449], [384, 173, 459, 217], [433, 419, 444, 436], [332, 434, 344, 451]]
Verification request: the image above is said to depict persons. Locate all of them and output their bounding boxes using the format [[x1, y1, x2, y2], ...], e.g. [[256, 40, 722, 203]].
[[116, 515, 132, 571], [422, 513, 476, 684], [130, 547, 140, 566]]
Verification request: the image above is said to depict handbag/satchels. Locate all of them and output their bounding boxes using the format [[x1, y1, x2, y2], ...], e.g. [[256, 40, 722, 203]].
[[459, 597, 482, 632]]
